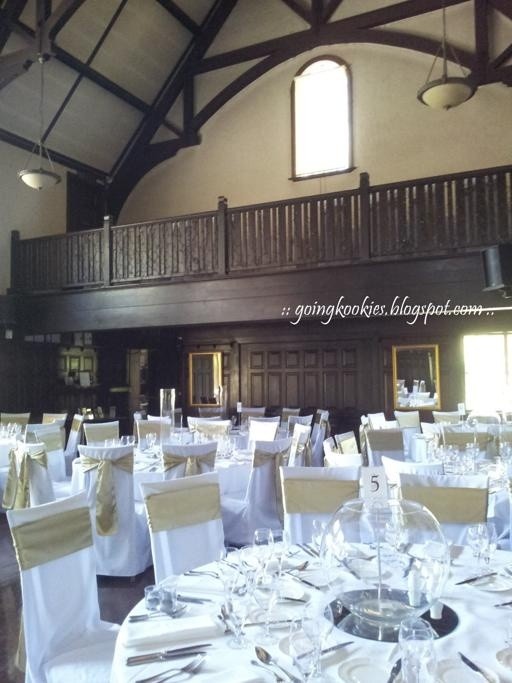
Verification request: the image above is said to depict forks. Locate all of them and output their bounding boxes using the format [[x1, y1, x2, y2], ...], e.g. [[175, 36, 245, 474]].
[[135, 654, 201, 683], [184, 569, 220, 578], [159, 659, 207, 683], [252, 660, 286, 683], [131, 606, 185, 626]]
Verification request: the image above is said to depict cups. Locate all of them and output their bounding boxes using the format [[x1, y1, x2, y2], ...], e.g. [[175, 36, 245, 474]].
[[161, 587, 176, 613], [396, 618, 431, 656], [219, 547, 242, 607], [469, 526, 488, 568], [253, 528, 271, 575], [146, 432, 157, 454], [480, 522, 497, 568], [104, 435, 135, 448], [254, 574, 280, 647], [290, 618, 322, 683], [400, 635, 437, 683], [303, 602, 337, 667], [241, 546, 264, 593], [269, 530, 286, 579], [225, 580, 252, 649], [144, 587, 159, 611]]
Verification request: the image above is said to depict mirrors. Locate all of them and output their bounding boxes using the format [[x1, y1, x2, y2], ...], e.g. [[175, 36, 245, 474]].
[[188, 350, 224, 408], [391, 344, 441, 411]]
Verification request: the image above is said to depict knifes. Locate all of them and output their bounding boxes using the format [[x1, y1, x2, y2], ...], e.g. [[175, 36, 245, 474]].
[[340, 561, 361, 580], [388, 660, 404, 682], [459, 651, 493, 682], [403, 559, 415, 579], [127, 650, 206, 667], [280, 594, 307, 603], [126, 643, 211, 667], [295, 640, 355, 664], [296, 543, 315, 556], [303, 542, 321, 557], [286, 572, 321, 592], [244, 618, 302, 628], [155, 586, 210, 607], [455, 572, 498, 585]]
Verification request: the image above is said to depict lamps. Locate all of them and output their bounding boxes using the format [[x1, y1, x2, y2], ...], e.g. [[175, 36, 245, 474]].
[[417, 0, 478, 111], [17, 53, 62, 190]]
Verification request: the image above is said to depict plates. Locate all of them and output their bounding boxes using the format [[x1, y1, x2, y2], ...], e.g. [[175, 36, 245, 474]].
[[282, 568, 335, 588], [407, 543, 450, 562], [342, 560, 389, 579], [280, 634, 338, 661], [256, 603, 305, 632], [430, 659, 501, 683], [468, 572, 512, 593], [338, 657, 395, 683]]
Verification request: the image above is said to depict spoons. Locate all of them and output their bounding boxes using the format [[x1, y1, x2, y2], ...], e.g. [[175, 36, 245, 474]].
[[256, 645, 302, 683]]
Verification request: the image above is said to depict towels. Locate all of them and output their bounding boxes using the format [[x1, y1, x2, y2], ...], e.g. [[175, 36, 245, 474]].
[[121, 615, 218, 650], [159, 573, 224, 597]]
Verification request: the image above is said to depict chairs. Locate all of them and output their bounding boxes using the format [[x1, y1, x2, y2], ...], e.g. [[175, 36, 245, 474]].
[[380, 456, 444, 502], [78, 445, 153, 583], [137, 419, 172, 450], [7, 488, 121, 683], [287, 415, 313, 438], [133, 412, 142, 443], [1, 413, 31, 432], [247, 421, 280, 450], [487, 425, 512, 456], [335, 431, 359, 455], [140, 471, 225, 585], [280, 465, 362, 545], [33, 426, 73, 495], [365, 428, 405, 466], [323, 444, 364, 468], [187, 416, 221, 434], [240, 407, 266, 427], [2, 440, 55, 509], [323, 437, 337, 456], [432, 411, 460, 424], [393, 410, 421, 434], [64, 413, 84, 477], [368, 413, 387, 429], [24, 423, 55, 444], [219, 437, 295, 545], [43, 413, 68, 424], [281, 408, 301, 428], [398, 473, 489, 545], [249, 417, 281, 434], [161, 441, 218, 479], [289, 423, 313, 466], [310, 408, 332, 467], [440, 424, 476, 446], [148, 415, 169, 424], [83, 420, 120, 448], [469, 410, 500, 425], [194, 420, 232, 443]]
